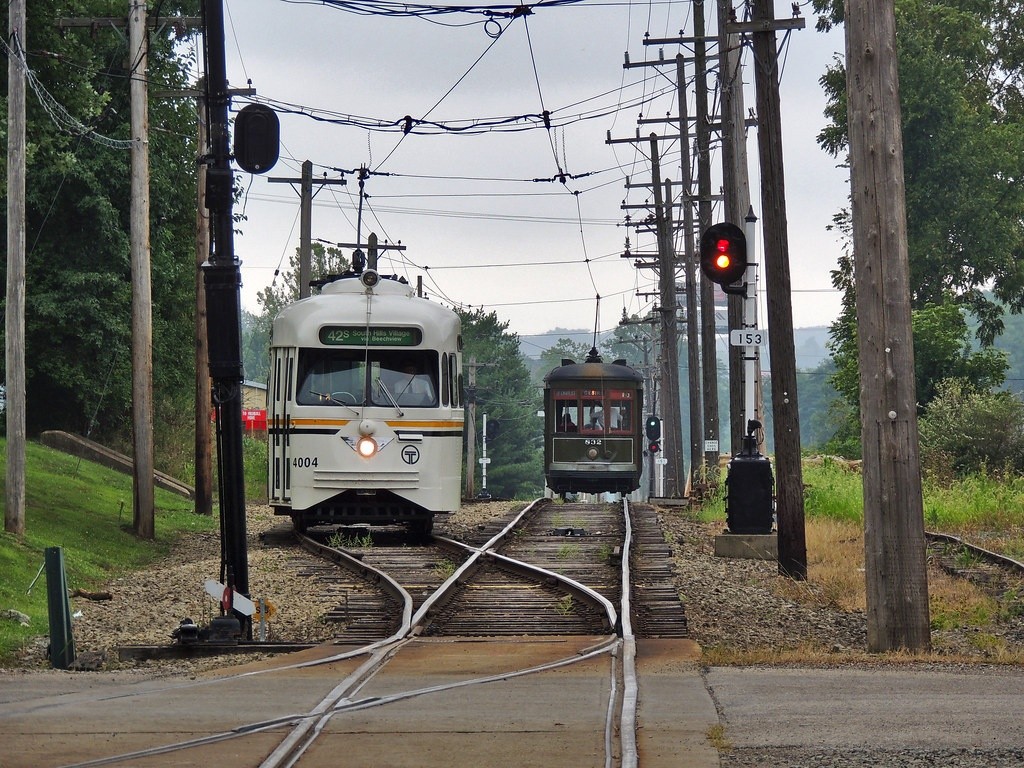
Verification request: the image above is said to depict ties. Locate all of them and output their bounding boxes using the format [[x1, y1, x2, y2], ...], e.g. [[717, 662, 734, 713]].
[[409, 382, 413, 394]]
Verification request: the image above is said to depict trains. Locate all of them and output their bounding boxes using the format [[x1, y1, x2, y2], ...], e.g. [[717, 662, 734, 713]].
[[265, 162, 465, 537], [542, 297, 645, 500]]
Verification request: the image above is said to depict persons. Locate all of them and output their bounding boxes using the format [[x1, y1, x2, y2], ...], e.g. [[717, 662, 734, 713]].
[[589, 399, 623, 432], [561, 413, 575, 426], [395, 361, 433, 401]]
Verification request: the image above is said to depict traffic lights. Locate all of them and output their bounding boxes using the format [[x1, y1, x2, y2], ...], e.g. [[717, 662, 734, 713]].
[[700, 223, 747, 284], [648, 442, 660, 453], [645, 416, 660, 441]]
